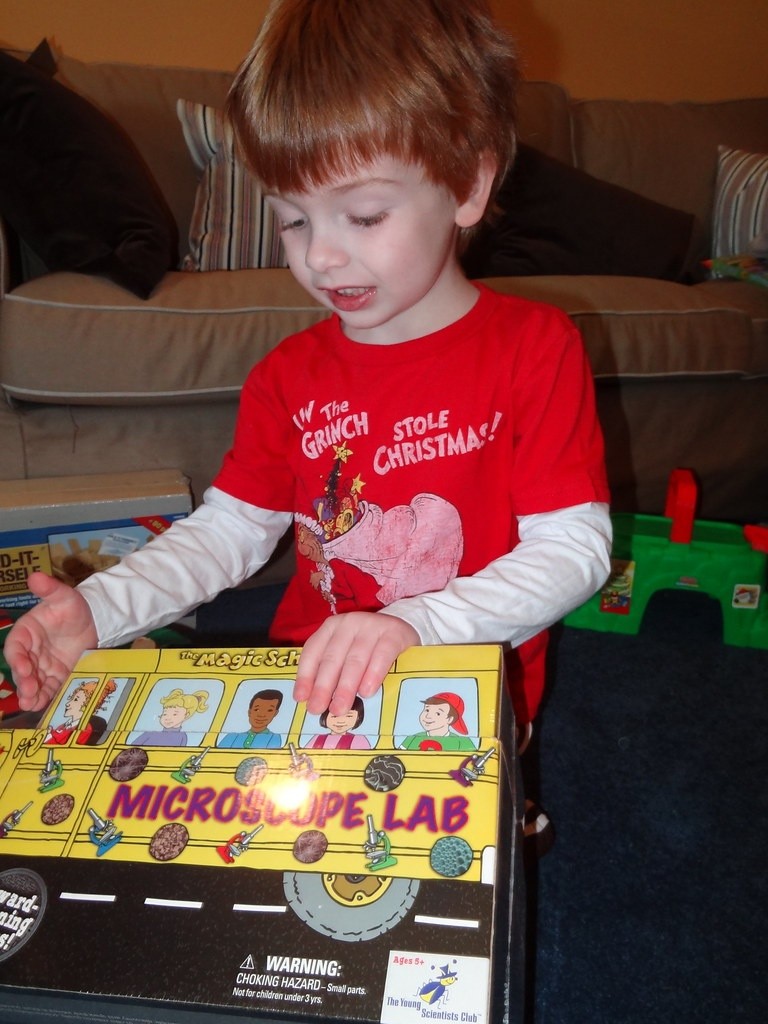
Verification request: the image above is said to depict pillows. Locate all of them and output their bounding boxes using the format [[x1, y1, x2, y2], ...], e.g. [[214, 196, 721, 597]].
[[714, 144, 768, 278], [176, 100, 292, 271]]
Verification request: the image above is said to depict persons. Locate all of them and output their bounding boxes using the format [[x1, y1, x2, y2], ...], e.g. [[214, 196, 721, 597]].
[[2, 0, 616, 862]]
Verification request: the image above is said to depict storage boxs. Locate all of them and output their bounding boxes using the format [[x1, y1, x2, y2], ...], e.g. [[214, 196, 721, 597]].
[[0, 644, 527, 1024], [0, 469, 192, 608]]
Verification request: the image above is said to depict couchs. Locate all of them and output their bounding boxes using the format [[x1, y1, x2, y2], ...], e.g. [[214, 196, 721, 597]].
[[0, 46, 768, 516]]
